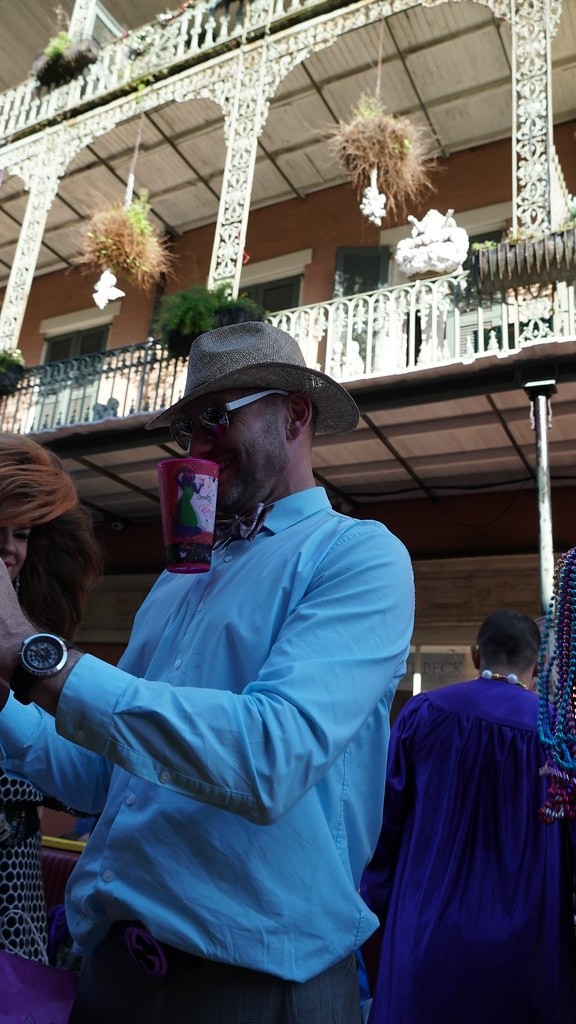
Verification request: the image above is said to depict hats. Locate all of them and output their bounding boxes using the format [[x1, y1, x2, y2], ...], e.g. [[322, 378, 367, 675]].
[[144, 320, 362, 437]]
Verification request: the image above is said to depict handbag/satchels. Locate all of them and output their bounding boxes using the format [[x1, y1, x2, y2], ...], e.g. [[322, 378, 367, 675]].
[[0, 910, 80, 1024]]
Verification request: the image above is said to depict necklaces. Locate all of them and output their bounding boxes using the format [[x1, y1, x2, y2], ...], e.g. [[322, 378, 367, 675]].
[[477, 669, 528, 690], [536, 545, 576, 823]]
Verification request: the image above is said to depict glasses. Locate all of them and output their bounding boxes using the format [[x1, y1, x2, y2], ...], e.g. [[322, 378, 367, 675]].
[[171, 389, 287, 450]]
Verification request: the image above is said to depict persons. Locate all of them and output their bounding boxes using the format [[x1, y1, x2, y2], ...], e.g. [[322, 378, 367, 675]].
[[2, 322, 420, 1024], [359, 605, 576, 1024], [1, 427, 100, 965]]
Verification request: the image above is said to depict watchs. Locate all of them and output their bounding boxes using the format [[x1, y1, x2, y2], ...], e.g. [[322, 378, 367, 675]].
[[11, 631, 74, 705]]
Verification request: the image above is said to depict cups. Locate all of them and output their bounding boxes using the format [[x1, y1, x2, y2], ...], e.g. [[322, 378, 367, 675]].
[[155, 457, 222, 574]]
